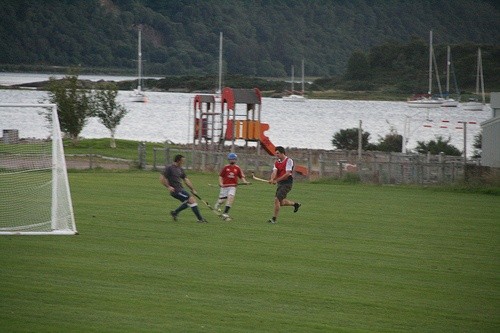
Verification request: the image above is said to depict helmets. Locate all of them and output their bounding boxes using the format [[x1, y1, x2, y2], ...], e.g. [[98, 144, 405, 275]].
[[227, 153, 237, 158]]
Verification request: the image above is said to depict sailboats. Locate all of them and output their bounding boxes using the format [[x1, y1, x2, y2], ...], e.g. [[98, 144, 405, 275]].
[[127, 30, 148, 103], [283, 60, 306, 102], [406, 30, 461, 108], [211, 32, 226, 103], [458, 47, 487, 111]]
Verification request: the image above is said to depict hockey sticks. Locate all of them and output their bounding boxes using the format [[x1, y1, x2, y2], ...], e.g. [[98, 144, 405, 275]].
[[207, 182, 252, 187], [251, 172, 276, 185], [190, 190, 224, 217]]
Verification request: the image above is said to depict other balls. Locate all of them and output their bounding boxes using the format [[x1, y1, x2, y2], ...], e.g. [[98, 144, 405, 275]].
[[218, 208, 222, 212]]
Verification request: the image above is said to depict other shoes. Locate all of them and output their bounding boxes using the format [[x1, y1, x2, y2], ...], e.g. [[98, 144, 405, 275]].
[[268, 218, 277, 223], [170, 210, 177, 221], [197, 218, 208, 222], [293, 201, 301, 212]]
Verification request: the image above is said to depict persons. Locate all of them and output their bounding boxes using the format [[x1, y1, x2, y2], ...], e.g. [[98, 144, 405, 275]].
[[215, 153, 247, 221], [161, 154, 208, 224], [267, 146, 302, 224]]
